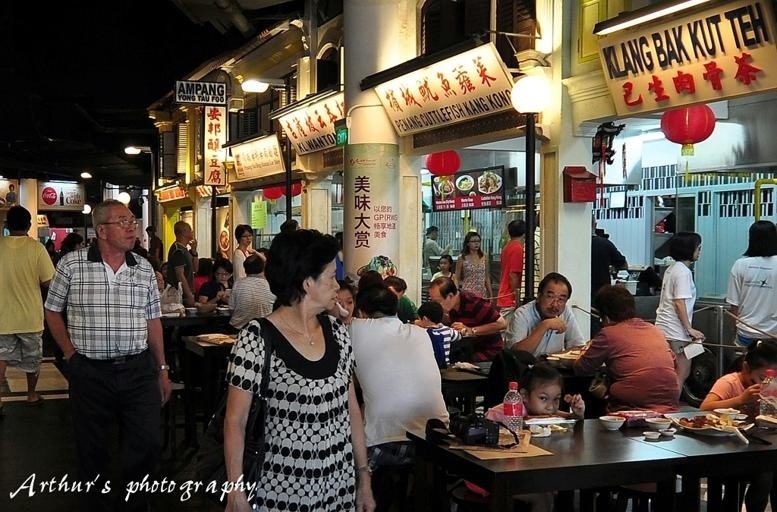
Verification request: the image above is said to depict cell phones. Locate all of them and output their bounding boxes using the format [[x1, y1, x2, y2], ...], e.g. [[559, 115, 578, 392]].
[[225, 289, 231, 294]]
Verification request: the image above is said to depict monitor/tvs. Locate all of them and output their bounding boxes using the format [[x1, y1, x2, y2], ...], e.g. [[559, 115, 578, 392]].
[[626, 266, 663, 287]]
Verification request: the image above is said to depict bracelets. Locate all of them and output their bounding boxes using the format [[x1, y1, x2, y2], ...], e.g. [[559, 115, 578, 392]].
[[353, 463, 374, 476]]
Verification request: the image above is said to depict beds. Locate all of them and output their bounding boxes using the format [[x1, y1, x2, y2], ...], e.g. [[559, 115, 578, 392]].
[[152, 275, 773, 511]]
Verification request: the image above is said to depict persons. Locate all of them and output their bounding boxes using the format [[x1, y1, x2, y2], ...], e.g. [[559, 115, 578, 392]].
[[5, 184, 16, 203], [592, 211, 631, 308], [576, 284, 680, 413], [702, 338, 774, 409], [724, 221, 777, 347], [656, 231, 701, 398], [220, 227, 379, 512], [1, 203, 55, 404]]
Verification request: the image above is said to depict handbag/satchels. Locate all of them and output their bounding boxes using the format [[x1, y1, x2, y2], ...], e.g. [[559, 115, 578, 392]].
[[203, 388, 270, 503]]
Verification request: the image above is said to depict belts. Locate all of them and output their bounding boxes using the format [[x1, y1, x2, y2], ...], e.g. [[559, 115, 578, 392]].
[[84, 349, 148, 366]]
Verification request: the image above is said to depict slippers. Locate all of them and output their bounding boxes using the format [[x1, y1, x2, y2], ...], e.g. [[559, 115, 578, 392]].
[[25, 397, 44, 407]]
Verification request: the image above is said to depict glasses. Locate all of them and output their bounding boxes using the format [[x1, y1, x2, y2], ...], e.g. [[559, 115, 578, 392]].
[[541, 293, 566, 306], [102, 220, 138, 230]]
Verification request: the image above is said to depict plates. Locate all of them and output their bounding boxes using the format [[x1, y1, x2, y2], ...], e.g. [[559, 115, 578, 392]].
[[218, 227, 230, 251], [662, 412, 755, 438], [477, 172, 502, 195]]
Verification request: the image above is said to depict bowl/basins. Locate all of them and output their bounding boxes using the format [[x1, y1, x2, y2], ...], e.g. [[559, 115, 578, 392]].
[[598, 415, 625, 431], [544, 345, 589, 366], [643, 415, 675, 441], [439, 182, 454, 198], [178, 301, 230, 315], [714, 406, 742, 420], [455, 174, 474, 194]]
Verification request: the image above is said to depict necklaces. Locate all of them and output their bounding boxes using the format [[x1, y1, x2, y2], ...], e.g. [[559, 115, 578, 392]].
[[277, 309, 320, 347]]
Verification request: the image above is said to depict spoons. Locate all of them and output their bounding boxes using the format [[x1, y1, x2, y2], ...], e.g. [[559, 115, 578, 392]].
[[724, 424, 750, 445], [432, 183, 441, 197], [336, 302, 349, 318]]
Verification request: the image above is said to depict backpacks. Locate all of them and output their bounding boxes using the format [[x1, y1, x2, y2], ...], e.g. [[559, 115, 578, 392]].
[[484, 348, 539, 411]]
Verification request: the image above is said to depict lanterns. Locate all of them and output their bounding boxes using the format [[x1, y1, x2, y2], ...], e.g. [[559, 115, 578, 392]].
[[281, 181, 302, 198], [659, 104, 717, 157], [263, 187, 282, 205], [425, 150, 462, 183]]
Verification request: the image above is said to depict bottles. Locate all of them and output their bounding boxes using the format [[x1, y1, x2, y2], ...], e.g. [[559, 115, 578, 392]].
[[759, 370, 777, 424], [504, 382, 523, 431], [59, 189, 64, 207]]
